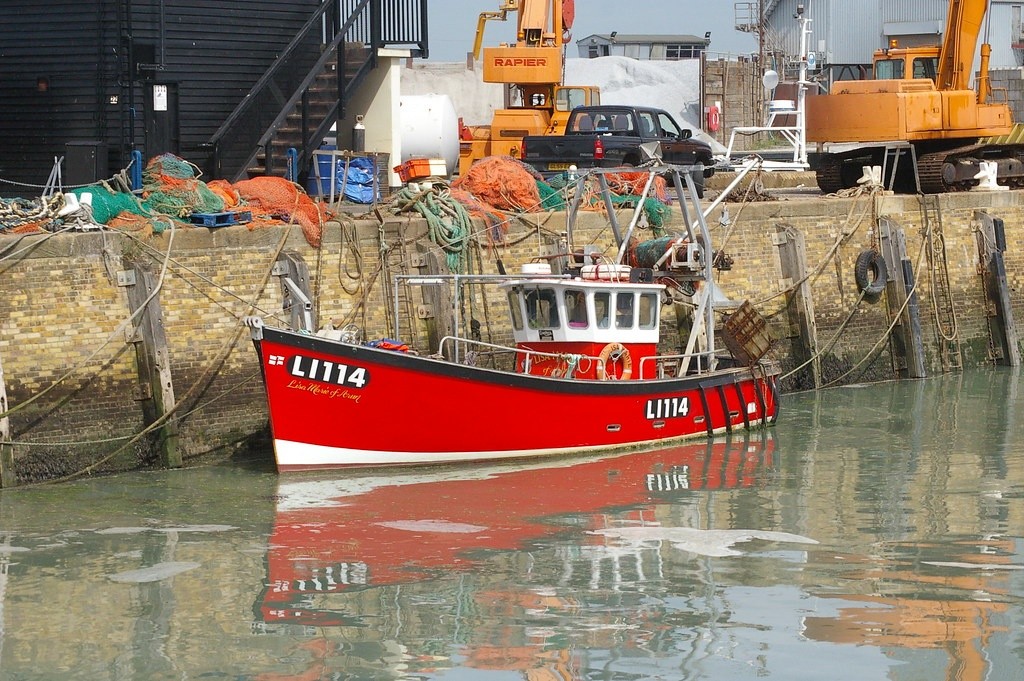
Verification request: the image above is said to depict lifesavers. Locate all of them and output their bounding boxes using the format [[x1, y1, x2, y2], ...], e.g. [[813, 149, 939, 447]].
[[582, 264, 631, 279], [596, 342, 632, 382], [855, 249, 889, 294]]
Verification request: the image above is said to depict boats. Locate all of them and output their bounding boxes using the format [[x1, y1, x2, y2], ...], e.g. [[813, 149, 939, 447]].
[[239, 185, 781, 472]]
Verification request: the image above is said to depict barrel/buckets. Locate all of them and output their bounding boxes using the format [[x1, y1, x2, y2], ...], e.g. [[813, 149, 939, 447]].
[[364, 152, 391, 199], [307, 145, 338, 196]]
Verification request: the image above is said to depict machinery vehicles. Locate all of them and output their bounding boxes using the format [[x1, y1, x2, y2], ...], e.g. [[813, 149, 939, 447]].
[[456, 0, 601, 183], [802, 1, 1024, 194]]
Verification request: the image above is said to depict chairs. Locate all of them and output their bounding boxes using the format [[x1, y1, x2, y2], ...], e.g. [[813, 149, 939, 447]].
[[615, 114, 628, 130], [640, 116, 650, 137], [578, 114, 593, 131]]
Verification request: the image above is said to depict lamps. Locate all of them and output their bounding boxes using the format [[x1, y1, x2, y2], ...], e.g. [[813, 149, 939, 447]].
[[610, 32, 617, 37], [705, 32, 711, 38]]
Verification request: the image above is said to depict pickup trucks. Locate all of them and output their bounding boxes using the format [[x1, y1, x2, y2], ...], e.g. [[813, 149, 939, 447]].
[[522, 105, 715, 199]]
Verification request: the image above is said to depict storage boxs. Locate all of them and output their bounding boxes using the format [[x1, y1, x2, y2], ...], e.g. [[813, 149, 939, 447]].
[[393, 158, 447, 181]]
